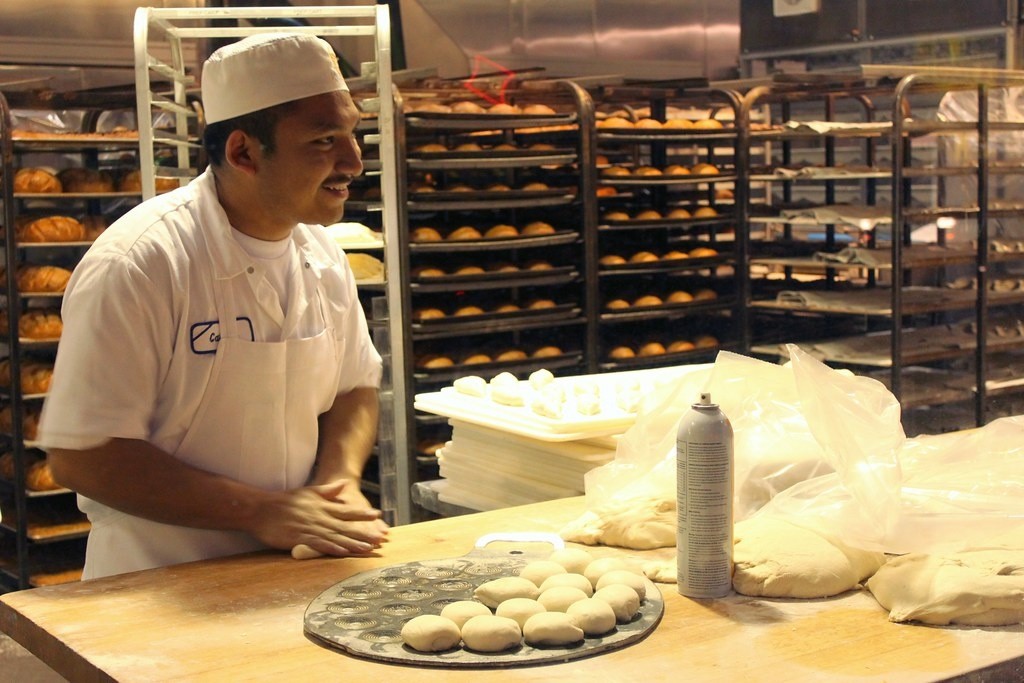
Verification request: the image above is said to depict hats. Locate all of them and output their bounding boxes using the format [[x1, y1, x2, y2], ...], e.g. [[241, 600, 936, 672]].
[[200, 32, 349, 126]]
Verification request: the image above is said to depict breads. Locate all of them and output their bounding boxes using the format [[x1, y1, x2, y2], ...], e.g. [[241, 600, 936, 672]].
[[1, 126, 178, 587], [326, 102, 726, 456]]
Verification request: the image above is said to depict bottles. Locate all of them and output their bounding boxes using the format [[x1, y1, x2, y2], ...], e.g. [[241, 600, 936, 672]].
[[675, 392, 735, 598]]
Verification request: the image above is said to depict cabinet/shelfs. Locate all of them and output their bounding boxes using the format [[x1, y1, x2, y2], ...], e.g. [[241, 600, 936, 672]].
[[1, 2, 408, 593], [396, 61, 1022, 482]]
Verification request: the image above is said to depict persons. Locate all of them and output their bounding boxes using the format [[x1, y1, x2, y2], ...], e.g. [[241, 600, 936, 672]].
[[33, 32, 392, 583]]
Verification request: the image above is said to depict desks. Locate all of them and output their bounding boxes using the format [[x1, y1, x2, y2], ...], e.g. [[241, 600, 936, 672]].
[[3, 415, 1020, 681]]
